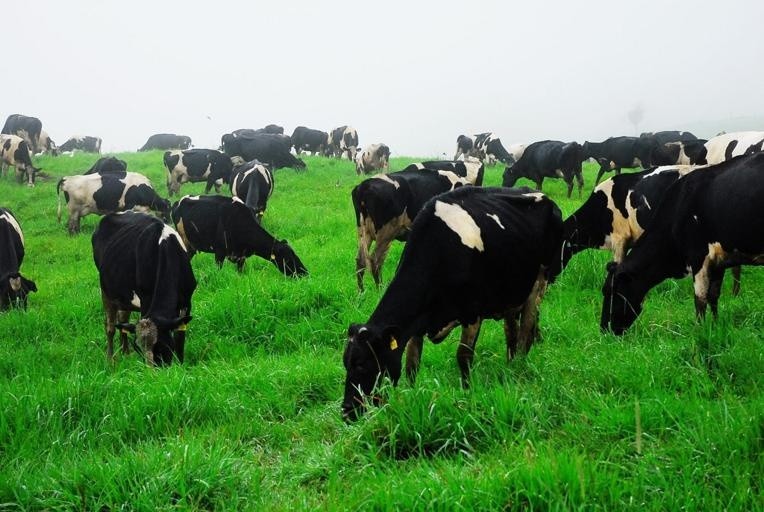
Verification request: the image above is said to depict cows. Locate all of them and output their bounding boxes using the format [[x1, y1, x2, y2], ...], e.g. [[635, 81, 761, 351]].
[[0, 134, 43, 186], [56, 172, 172, 237], [342, 186, 562, 426], [137, 133, 193, 151], [91, 210, 198, 370], [402, 161, 484, 185], [230, 159, 274, 224], [170, 192, 306, 278], [220, 124, 357, 172], [29, 114, 59, 154], [57, 135, 102, 154], [351, 168, 474, 288], [164, 150, 235, 199], [0, 114, 43, 157], [502, 128, 764, 337], [454, 133, 515, 172], [0, 208, 40, 314], [359, 143, 392, 177]]
